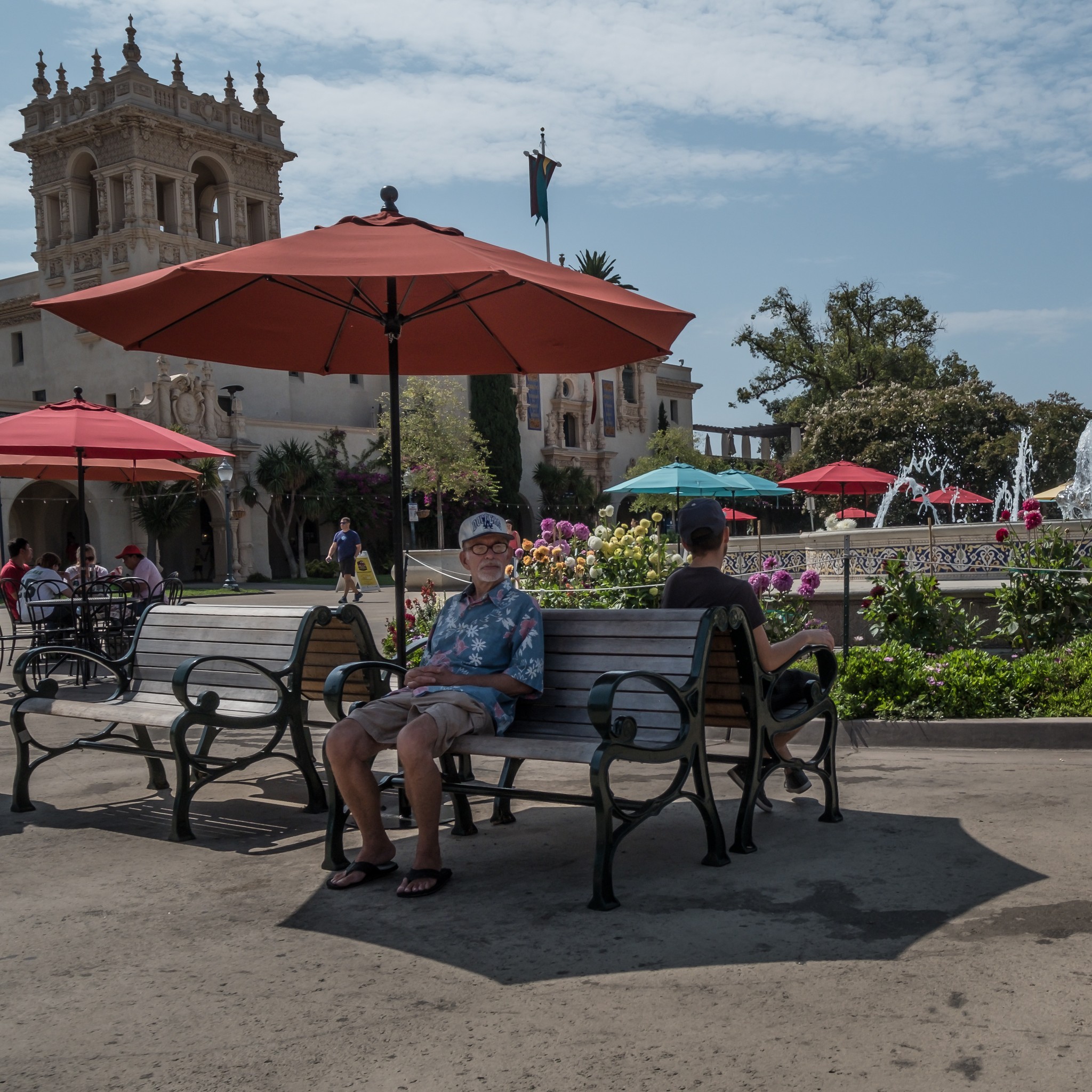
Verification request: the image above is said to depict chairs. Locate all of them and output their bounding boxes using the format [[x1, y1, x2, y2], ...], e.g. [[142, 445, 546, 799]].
[[0, 571, 184, 685]]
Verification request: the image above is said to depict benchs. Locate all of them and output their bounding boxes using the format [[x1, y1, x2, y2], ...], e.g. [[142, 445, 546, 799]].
[[9, 604, 845, 911]]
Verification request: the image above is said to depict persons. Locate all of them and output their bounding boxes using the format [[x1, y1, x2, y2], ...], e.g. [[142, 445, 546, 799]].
[[115, 544, 169, 638], [326, 517, 363, 603], [326, 511, 545, 898], [63, 544, 113, 626], [0, 537, 78, 659], [661, 497, 835, 812], [504, 519, 524, 590]]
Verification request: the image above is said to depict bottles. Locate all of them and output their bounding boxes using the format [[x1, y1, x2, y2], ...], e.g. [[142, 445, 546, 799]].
[[73, 579, 79, 598], [88, 564, 97, 591]]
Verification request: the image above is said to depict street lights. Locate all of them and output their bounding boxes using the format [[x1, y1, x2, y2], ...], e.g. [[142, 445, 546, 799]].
[[215, 456, 242, 593], [403, 466, 417, 550]]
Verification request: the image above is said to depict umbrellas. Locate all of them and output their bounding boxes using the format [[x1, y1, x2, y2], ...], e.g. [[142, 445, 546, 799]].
[[911, 485, 994, 504], [1032, 482, 1067, 503], [777, 454, 927, 521], [602, 454, 795, 556], [0, 385, 237, 599], [30, 187, 696, 696]]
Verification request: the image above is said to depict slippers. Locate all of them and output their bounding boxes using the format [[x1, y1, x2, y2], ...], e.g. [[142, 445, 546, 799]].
[[326, 859, 399, 889], [396, 866, 453, 897]]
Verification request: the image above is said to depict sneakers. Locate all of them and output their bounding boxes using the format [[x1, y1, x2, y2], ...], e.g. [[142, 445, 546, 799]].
[[352, 592, 363, 601], [727, 763, 772, 811], [338, 596, 348, 603], [783, 767, 812, 793]]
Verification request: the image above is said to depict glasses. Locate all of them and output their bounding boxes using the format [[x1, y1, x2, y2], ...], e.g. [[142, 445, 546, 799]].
[[462, 543, 510, 555], [340, 522, 349, 525], [122, 556, 130, 563], [85, 556, 95, 562]]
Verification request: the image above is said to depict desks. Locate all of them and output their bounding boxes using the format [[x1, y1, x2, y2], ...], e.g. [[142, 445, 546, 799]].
[[74, 589, 131, 598], [25, 597, 140, 681]]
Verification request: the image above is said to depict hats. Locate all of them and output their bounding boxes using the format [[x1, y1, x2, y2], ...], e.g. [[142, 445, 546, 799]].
[[115, 545, 141, 559], [677, 498, 726, 547], [459, 513, 514, 548]]
